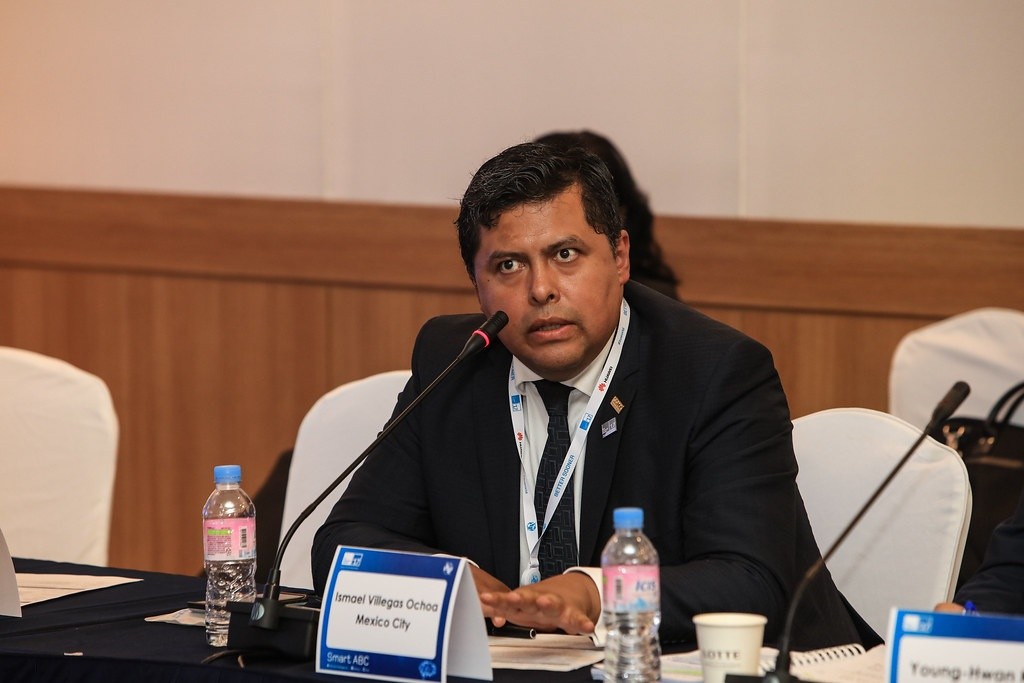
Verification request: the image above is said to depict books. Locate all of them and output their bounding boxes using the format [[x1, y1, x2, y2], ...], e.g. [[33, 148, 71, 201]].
[[591, 643, 888, 682]]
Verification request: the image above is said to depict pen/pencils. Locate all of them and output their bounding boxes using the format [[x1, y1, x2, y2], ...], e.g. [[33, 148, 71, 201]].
[[486, 623, 537, 639]]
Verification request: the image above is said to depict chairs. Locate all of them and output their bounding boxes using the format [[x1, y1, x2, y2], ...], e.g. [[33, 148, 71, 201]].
[[791, 408, 971, 643], [887, 305, 1024, 438], [0, 346, 122, 565], [279, 369, 412, 601]]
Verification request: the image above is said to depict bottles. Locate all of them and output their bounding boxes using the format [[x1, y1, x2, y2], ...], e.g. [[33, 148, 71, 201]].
[[202, 465, 257, 649], [601, 507, 663, 683]]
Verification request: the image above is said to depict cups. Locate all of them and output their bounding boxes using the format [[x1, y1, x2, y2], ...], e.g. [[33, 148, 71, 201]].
[[692, 611, 768, 683]]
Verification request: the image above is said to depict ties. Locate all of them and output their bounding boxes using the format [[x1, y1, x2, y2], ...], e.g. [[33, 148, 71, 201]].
[[530, 378, 578, 581]]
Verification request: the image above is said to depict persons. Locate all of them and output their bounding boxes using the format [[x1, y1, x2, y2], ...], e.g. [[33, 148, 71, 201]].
[[930, 499, 1024, 621], [311, 137, 865, 650]]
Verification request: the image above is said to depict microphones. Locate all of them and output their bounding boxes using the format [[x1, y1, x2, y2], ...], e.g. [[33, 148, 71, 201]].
[[226, 310, 509, 660], [726, 381, 971, 683]]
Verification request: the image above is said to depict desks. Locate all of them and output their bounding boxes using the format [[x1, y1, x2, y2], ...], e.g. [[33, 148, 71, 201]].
[[0, 559, 700, 683]]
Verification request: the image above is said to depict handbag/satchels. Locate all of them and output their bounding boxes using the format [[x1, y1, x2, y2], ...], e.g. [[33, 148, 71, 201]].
[[938, 383, 1024, 596]]
